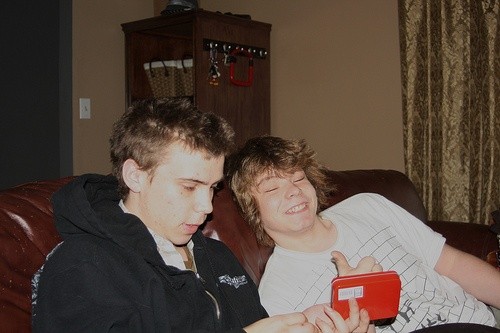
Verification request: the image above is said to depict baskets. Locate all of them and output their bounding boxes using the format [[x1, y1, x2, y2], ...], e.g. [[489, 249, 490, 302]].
[[144, 57, 195, 99]]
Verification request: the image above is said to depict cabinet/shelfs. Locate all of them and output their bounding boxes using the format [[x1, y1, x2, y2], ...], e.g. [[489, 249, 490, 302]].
[[121, 8, 272, 150]]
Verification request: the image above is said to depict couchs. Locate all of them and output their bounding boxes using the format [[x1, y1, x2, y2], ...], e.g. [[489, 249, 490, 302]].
[[0, 169, 500, 333]]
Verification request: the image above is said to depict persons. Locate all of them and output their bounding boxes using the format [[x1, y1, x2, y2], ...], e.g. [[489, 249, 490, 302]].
[[30, 95, 377, 333], [227, 135, 499, 332]]
